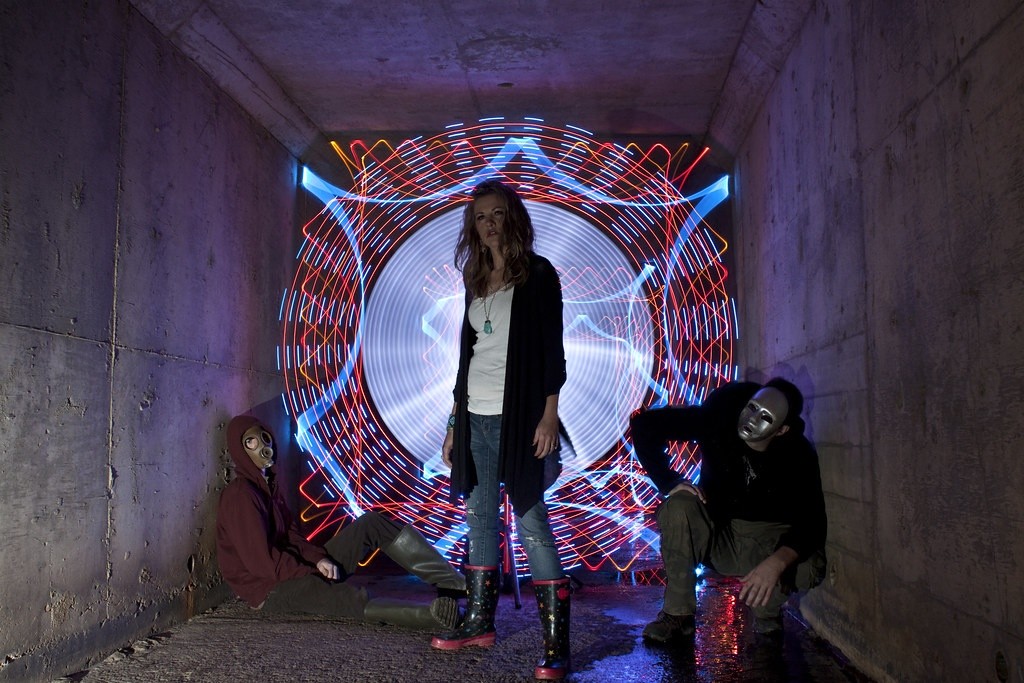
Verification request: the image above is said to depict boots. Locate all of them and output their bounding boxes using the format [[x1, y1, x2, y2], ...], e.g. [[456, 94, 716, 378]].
[[365, 597, 461, 632], [431, 564, 498, 650], [382, 522, 503, 599], [532, 578, 571, 680]]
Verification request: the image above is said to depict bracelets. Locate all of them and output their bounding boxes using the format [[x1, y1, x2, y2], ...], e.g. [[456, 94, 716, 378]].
[[445, 414, 455, 431]]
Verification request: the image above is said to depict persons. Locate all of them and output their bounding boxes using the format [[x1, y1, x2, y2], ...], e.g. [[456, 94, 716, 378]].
[[629, 375, 829, 644], [216, 414, 466, 631], [429, 179, 579, 681]]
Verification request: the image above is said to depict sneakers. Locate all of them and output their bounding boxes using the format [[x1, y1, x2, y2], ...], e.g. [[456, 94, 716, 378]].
[[746, 618, 778, 636], [641, 610, 697, 643]]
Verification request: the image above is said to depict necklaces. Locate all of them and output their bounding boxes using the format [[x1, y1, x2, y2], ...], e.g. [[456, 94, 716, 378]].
[[483, 270, 504, 335]]
